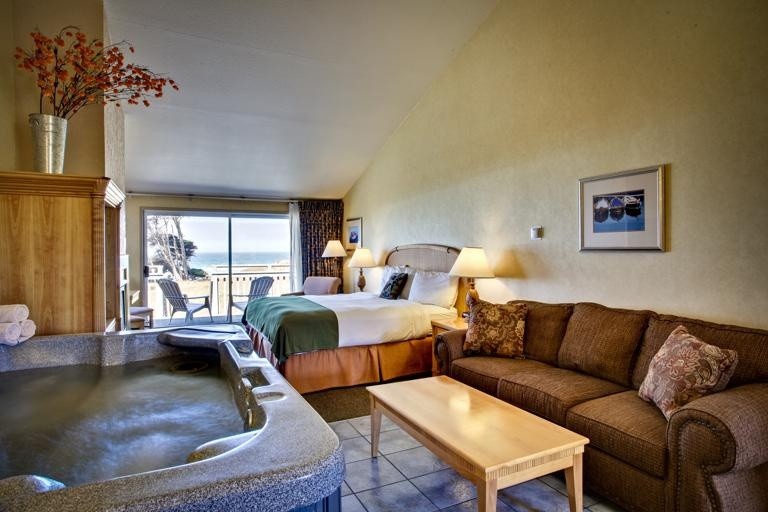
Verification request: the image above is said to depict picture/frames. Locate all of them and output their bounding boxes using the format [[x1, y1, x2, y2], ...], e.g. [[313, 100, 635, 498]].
[[346, 217, 362, 251], [578, 164, 665, 252]]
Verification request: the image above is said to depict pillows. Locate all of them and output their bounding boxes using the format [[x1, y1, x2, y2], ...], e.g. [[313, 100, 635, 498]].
[[638, 325, 740, 423], [378, 265, 416, 299], [408, 271, 459, 307], [463, 298, 528, 360], [558, 302, 651, 387], [379, 273, 408, 299], [632, 310, 768, 389], [508, 301, 573, 365]]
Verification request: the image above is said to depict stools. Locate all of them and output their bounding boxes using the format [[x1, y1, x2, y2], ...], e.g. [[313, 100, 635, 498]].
[[131, 316, 145, 330], [131, 307, 154, 328]]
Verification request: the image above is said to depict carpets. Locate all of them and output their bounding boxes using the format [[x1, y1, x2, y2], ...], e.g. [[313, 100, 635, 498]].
[[302, 373, 430, 422]]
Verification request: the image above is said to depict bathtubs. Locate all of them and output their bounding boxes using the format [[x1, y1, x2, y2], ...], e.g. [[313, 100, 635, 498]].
[[1, 323, 348, 512]]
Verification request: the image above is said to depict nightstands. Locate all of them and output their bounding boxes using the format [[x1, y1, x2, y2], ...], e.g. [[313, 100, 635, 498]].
[[431, 318, 468, 377]]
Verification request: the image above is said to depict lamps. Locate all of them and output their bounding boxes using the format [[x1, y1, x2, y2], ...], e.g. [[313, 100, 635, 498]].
[[449, 248, 495, 322], [350, 249, 377, 292], [322, 241, 347, 278]]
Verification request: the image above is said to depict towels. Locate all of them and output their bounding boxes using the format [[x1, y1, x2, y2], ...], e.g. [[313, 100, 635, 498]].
[[21, 320, 36, 343], [0, 304, 29, 322], [0, 323, 22, 344]]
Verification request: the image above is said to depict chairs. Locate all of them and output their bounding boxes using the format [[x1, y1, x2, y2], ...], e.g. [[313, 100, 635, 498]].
[[156, 278, 213, 325], [227, 276, 274, 320], [281, 276, 341, 295]]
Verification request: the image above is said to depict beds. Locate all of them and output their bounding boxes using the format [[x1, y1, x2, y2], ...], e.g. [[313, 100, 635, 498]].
[[241, 243, 473, 395]]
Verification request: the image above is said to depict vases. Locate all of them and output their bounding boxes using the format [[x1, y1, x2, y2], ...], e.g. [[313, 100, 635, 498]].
[[27, 114, 68, 175]]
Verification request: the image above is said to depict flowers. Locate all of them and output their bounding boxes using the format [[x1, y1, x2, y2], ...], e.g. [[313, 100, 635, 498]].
[[14, 25, 180, 119]]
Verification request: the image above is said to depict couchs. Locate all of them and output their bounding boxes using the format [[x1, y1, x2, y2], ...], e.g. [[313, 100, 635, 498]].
[[434, 301, 768, 511]]
